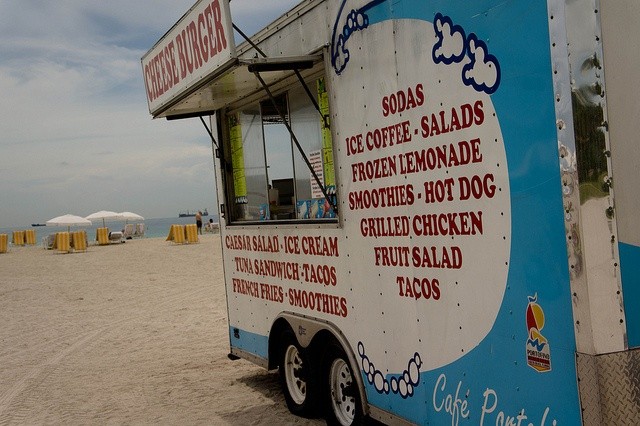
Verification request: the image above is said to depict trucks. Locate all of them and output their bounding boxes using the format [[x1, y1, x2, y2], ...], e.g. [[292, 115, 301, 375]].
[[141, 0, 639, 425]]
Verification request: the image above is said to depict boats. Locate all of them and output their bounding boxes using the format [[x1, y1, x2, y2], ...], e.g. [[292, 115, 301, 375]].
[[32, 223, 45, 226]]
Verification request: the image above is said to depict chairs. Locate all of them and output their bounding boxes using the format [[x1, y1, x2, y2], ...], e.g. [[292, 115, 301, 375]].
[[40, 233, 57, 250], [122, 222, 134, 241], [13, 230, 24, 246], [184, 223, 199, 246], [69, 230, 88, 252], [0, 233, 9, 253], [205, 224, 218, 232], [53, 231, 68, 255], [165, 224, 185, 245], [133, 223, 146, 239], [94, 228, 108, 245], [25, 228, 34, 245], [108, 229, 123, 243]]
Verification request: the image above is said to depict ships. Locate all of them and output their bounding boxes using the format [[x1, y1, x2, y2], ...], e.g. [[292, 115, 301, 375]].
[[179, 207, 207, 216]]
